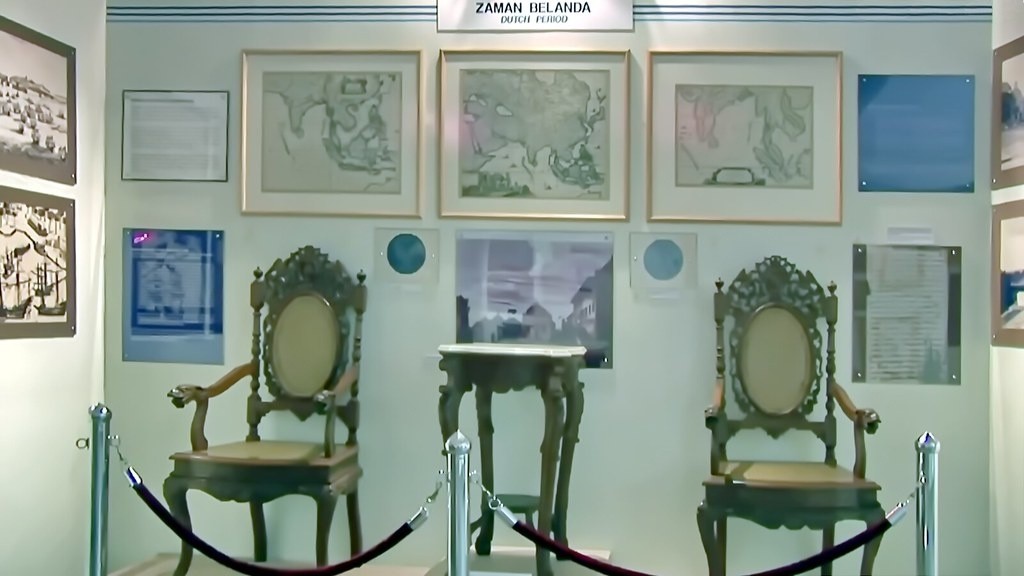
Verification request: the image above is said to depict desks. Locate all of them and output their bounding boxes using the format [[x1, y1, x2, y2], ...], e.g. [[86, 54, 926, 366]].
[[438, 342, 587, 576]]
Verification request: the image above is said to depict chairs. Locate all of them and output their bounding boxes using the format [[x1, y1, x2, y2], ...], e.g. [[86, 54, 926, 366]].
[[697, 256, 886, 576], [163, 245, 367, 576]]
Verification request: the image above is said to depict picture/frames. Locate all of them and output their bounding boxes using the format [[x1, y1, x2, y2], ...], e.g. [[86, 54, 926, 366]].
[[647, 50, 843, 225], [992, 199, 1024, 349], [0, 15, 77, 186], [0, 185, 77, 339], [438, 48, 629, 221], [240, 50, 423, 221], [993, 35, 1024, 190]]
